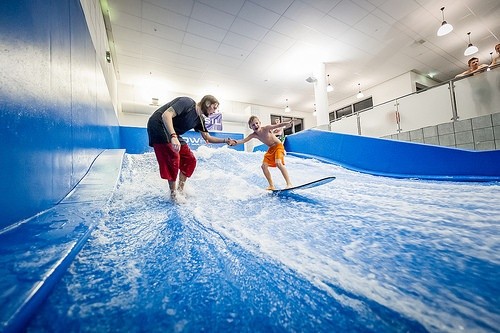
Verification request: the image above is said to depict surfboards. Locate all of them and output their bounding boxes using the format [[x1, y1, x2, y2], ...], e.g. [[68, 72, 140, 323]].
[[273, 175, 336, 192]]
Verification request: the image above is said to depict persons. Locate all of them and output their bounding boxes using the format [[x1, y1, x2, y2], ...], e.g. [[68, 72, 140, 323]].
[[227, 116, 292, 189], [489, 44, 500, 66], [147, 93, 235, 195], [455, 57, 488, 77]]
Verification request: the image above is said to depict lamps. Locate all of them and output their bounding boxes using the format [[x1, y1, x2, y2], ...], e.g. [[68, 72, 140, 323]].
[[437, 7, 453, 36], [464, 32, 478, 56], [357, 83, 364, 99], [326, 74, 332, 91]]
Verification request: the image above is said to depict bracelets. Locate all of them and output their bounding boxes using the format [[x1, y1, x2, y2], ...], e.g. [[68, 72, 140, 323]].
[[170, 133, 177, 138]]
